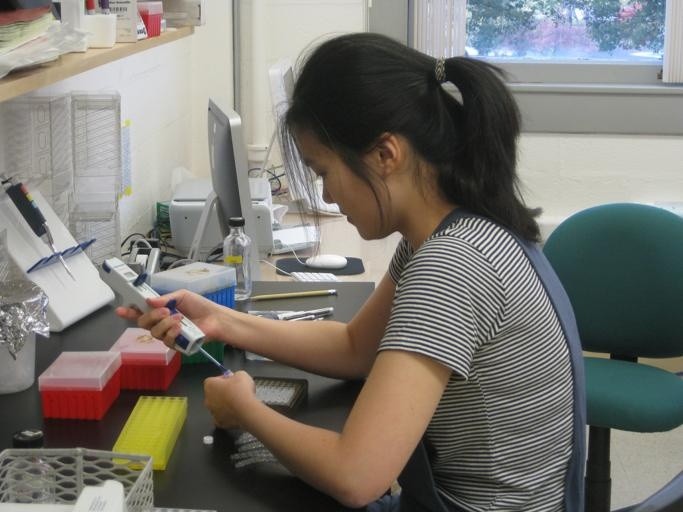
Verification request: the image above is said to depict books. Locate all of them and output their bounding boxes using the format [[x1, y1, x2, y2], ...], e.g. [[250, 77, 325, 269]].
[[0, 1, 56, 54]]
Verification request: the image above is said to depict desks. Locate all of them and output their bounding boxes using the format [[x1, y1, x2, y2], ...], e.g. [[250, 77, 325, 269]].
[[0, 282, 375, 512]]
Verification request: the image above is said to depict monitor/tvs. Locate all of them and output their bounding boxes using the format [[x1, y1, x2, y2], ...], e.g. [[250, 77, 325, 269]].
[[162, 96, 256, 269], [255, 60, 317, 214]]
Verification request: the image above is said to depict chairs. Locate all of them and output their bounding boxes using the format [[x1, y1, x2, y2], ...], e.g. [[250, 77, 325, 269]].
[[541, 204, 681, 512]]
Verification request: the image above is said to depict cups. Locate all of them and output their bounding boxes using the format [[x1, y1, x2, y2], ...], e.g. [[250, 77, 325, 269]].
[[0, 331, 42, 395]]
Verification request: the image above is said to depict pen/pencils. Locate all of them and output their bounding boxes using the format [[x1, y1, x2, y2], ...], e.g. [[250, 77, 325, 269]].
[[250, 289, 336, 299]]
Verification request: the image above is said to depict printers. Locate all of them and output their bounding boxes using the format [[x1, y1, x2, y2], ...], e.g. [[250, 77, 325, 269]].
[[168, 177, 318, 261]]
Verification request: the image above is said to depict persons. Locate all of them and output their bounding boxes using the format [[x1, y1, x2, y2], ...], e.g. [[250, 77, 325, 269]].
[[111, 28, 591, 512]]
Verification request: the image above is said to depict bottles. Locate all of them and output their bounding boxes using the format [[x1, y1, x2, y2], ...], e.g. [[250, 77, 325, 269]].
[[221, 215, 253, 302]]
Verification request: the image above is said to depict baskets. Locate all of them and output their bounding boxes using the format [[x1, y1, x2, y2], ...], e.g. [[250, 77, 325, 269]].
[[0, 447, 154, 512]]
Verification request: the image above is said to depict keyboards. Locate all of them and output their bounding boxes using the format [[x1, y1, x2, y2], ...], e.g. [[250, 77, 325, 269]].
[[290, 271, 337, 282], [308, 176, 342, 216]]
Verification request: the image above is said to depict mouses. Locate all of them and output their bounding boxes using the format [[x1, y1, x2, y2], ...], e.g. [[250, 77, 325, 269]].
[[305, 254, 348, 269]]
